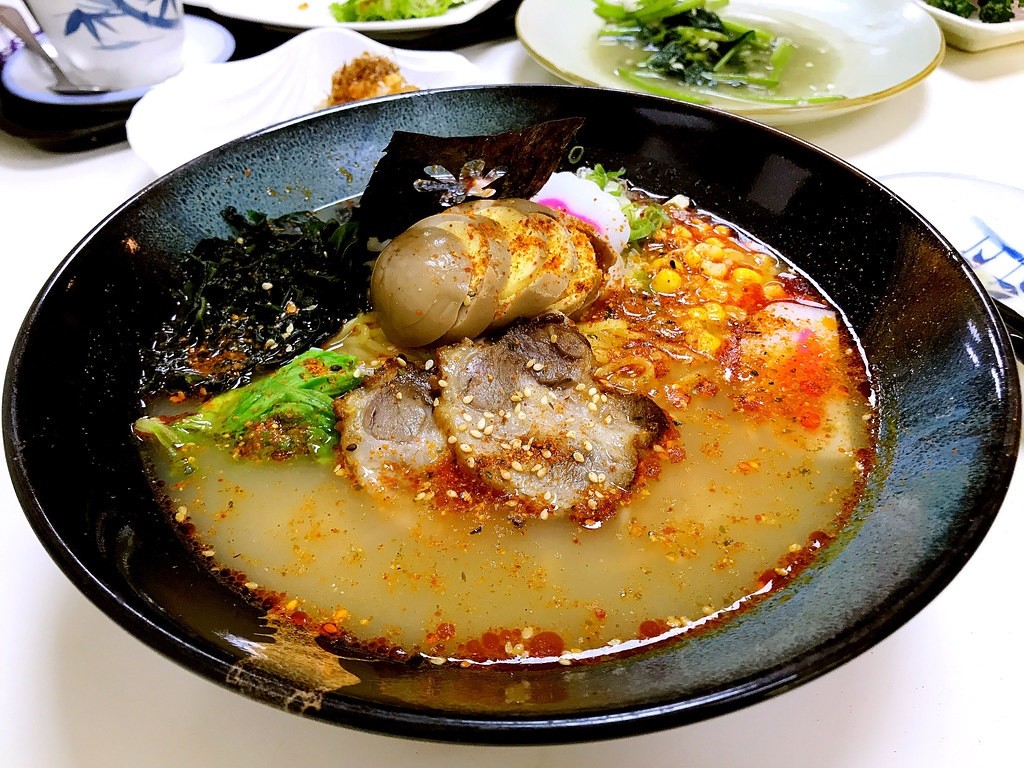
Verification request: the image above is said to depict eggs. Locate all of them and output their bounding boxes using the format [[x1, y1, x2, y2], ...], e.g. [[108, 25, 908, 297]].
[[368, 197, 613, 350]]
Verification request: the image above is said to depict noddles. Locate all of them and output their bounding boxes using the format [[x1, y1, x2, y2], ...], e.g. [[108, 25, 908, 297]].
[[323, 305, 710, 404]]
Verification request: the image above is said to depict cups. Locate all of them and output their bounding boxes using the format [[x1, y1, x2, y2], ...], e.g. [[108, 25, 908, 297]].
[[26, 0, 184, 91]]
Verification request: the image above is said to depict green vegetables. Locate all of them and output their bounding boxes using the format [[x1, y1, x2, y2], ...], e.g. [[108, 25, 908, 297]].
[[589, 0, 845, 103]]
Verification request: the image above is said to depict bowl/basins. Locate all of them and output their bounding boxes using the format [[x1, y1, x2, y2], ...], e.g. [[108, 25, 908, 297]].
[[2, 83, 1022, 748]]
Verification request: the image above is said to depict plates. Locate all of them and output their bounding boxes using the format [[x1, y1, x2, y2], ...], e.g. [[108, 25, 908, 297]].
[[1, 14, 236, 105], [184, 0, 500, 40], [514, 0, 947, 126], [913, 0, 1024, 53], [125, 27, 481, 178]]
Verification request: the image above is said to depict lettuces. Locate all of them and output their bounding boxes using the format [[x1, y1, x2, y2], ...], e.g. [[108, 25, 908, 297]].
[[127, 347, 369, 479], [329, 0, 467, 22]]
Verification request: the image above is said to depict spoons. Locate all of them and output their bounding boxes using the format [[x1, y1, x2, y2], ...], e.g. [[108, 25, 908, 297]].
[[0, 4, 111, 96]]
